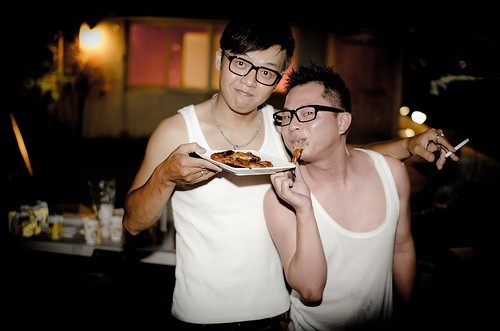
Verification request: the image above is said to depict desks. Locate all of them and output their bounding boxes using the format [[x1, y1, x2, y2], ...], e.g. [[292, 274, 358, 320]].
[[25, 235, 175, 284]]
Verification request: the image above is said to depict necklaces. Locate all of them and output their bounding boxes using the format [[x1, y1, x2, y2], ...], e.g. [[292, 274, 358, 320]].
[[211, 98, 262, 150]]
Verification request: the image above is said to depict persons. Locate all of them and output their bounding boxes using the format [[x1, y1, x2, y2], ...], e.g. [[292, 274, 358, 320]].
[[263, 64, 417, 331], [122, 12, 460, 331]]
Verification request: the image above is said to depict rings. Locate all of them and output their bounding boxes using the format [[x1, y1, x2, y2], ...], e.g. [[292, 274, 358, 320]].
[[435, 134, 445, 142], [201, 171, 204, 176]]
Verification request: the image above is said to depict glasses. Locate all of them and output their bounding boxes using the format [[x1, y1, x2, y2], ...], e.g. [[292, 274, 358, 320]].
[[222, 51, 282, 86], [273, 104, 344, 126]]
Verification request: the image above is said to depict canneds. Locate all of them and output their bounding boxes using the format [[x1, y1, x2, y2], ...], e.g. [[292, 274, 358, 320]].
[[50, 214, 64, 240]]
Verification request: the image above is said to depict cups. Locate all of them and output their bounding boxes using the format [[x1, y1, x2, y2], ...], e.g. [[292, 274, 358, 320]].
[[81, 204, 125, 245], [8, 202, 65, 240]]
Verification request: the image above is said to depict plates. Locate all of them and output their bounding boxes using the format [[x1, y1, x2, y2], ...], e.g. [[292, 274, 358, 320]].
[[194, 149, 296, 176]]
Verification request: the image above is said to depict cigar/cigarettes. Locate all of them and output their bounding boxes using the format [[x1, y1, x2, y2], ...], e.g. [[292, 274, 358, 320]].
[[445, 138, 469, 158]]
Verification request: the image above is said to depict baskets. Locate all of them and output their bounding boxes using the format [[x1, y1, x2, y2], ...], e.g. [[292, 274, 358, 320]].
[[41, 216, 82, 239], [82, 208, 174, 252]]
